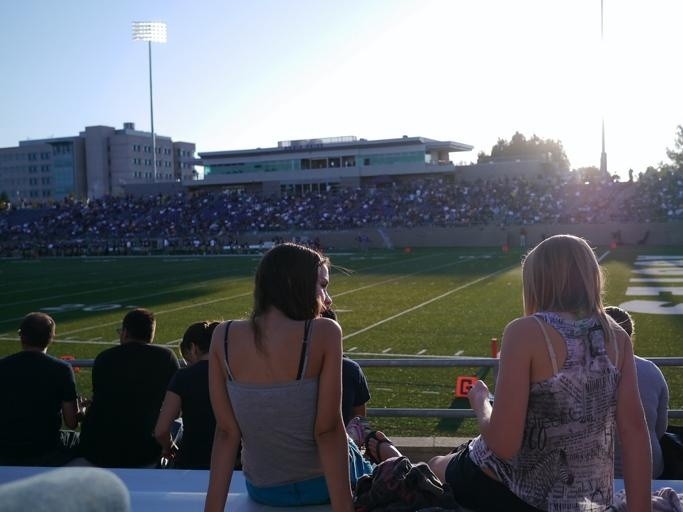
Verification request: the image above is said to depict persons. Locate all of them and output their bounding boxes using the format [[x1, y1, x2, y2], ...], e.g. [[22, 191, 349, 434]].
[[1, 167, 682, 260], [318, 306, 370, 431], [0, 310, 84, 465], [363, 232, 653, 512], [602, 305, 671, 481], [80, 307, 179, 468], [151, 320, 242, 470], [203, 241, 375, 511]]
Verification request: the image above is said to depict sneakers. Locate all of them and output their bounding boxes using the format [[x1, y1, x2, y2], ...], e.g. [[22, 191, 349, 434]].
[[345, 412, 367, 452]]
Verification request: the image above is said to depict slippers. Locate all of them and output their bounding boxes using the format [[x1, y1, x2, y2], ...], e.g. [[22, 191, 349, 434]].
[[366, 430, 391, 466]]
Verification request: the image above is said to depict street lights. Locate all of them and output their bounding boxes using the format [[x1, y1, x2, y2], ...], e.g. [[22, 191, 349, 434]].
[[131, 20, 168, 180]]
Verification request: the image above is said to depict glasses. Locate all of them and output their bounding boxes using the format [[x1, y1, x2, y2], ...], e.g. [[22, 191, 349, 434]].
[[116, 327, 123, 334], [16, 328, 23, 336]]
